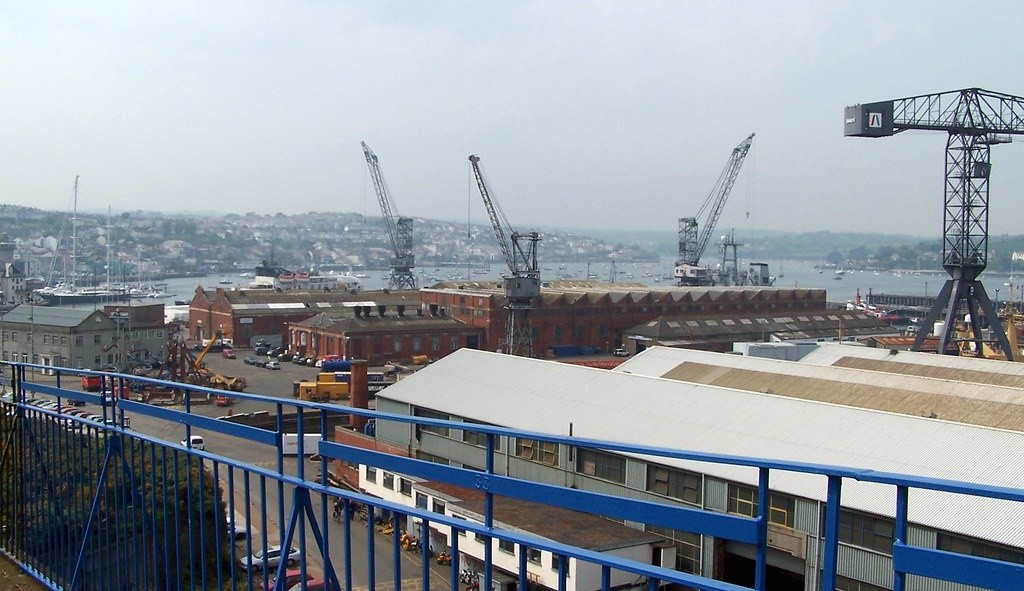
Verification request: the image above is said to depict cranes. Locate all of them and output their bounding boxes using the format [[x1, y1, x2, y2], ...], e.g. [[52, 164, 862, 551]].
[[361, 141, 415, 289], [468, 154, 542, 359]]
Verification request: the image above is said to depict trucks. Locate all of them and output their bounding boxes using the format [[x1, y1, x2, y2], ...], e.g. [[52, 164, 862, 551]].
[[222, 349, 235, 358], [107, 386, 129, 399], [82, 375, 100, 392]]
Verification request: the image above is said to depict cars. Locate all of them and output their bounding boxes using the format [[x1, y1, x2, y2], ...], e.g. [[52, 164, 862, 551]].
[[239, 545, 300, 573], [244, 356, 280, 370], [227, 517, 247, 543], [67, 398, 85, 406], [255, 339, 322, 368], [261, 569, 331, 591], [3, 393, 130, 438]]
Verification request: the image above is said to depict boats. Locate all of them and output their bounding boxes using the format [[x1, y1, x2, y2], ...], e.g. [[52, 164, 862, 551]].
[[31, 175, 175, 305], [220, 258, 369, 291]]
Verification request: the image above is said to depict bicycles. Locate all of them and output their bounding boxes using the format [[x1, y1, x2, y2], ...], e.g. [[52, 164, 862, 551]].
[[331, 507, 341, 523]]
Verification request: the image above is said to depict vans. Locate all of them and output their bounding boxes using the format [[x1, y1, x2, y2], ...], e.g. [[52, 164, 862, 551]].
[[100, 391, 117, 405]]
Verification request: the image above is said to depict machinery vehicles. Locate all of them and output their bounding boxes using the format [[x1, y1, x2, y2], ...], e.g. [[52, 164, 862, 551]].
[[674, 132, 755, 286], [187, 329, 231, 374]]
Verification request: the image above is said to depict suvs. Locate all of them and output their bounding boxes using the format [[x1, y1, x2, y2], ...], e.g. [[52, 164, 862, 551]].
[[180, 436, 204, 451], [613, 349, 629, 357], [77, 368, 91, 377]]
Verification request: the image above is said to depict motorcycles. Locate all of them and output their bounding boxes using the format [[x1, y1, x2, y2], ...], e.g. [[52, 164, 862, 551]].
[[411, 536, 433, 558]]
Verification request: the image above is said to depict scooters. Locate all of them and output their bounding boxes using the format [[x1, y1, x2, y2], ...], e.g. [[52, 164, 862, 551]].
[[437, 546, 452, 565], [459, 568, 479, 591]]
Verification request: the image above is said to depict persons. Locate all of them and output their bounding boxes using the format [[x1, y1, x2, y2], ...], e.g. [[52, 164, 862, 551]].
[[334, 496, 355, 521]]
